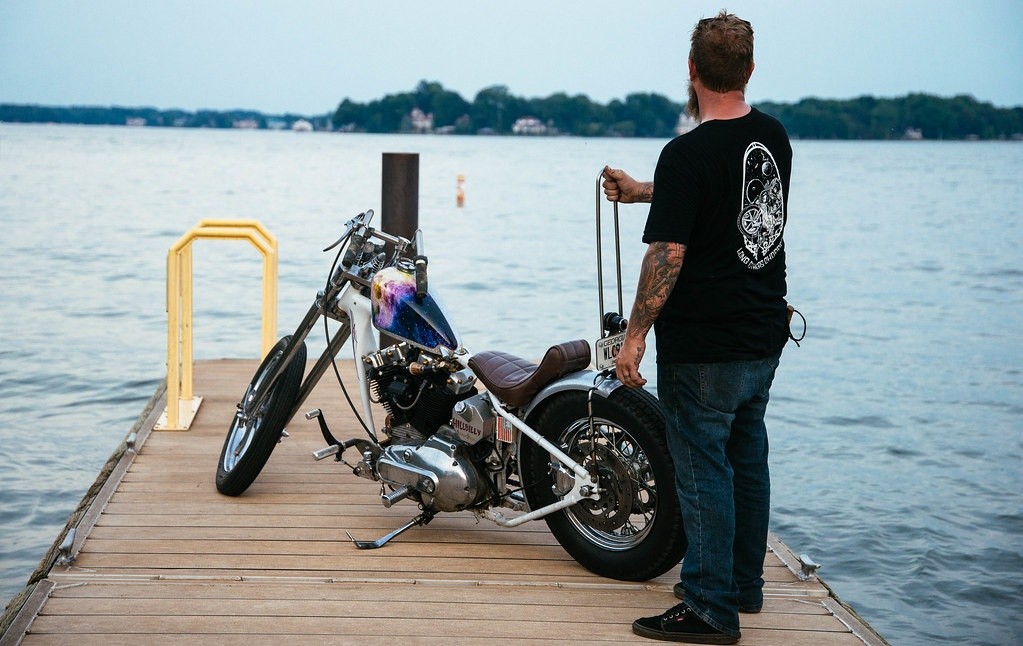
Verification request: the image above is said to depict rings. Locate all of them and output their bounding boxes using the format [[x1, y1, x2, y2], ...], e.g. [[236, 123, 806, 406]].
[[624, 375, 631, 382]]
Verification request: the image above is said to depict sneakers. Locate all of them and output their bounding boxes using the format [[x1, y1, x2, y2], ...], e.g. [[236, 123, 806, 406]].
[[674, 582, 762, 614], [633, 602, 739, 644]]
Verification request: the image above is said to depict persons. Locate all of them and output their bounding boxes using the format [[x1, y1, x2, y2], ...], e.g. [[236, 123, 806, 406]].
[[602, 9, 793, 645]]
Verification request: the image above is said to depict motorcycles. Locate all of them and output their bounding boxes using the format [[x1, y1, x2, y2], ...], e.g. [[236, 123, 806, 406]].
[[215, 166, 687, 582]]
